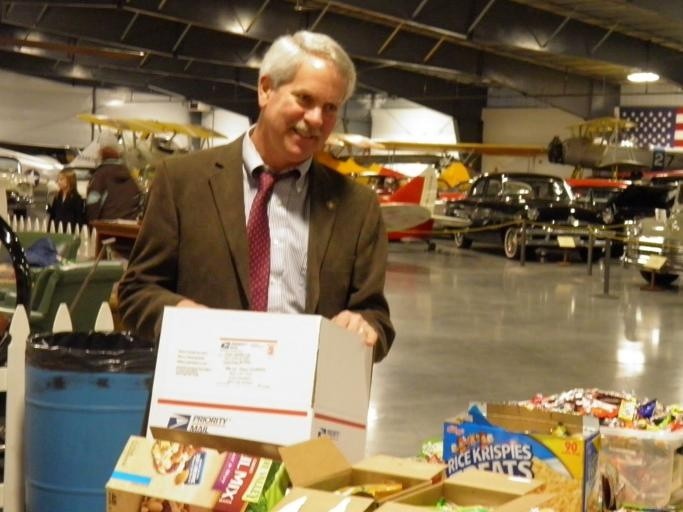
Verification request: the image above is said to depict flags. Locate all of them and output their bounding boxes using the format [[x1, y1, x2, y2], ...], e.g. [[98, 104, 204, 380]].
[[614, 105, 683, 153]]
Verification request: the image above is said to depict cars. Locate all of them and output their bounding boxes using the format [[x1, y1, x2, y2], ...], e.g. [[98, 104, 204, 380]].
[[445, 170, 610, 263], [0, 147, 65, 223], [626, 186, 683, 288], [564, 176, 630, 213]]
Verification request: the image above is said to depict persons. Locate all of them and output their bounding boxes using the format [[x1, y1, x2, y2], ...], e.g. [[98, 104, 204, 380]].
[[119, 31, 395, 363], [85, 147, 141, 223], [47, 169, 88, 234]]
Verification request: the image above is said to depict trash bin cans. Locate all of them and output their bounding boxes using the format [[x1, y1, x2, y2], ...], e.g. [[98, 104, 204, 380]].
[[26, 331, 158, 511]]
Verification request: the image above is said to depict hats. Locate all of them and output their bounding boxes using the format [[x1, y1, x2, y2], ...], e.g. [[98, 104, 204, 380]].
[[103, 148, 118, 160]]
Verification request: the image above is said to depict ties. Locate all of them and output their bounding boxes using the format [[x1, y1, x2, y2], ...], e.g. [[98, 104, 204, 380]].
[[248, 170, 299, 312]]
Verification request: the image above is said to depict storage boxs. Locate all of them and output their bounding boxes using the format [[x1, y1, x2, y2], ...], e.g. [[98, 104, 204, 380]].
[[103, 303, 682, 510]]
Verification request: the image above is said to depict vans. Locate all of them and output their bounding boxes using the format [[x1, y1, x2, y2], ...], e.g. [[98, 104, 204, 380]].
[[603, 182, 683, 246]]
